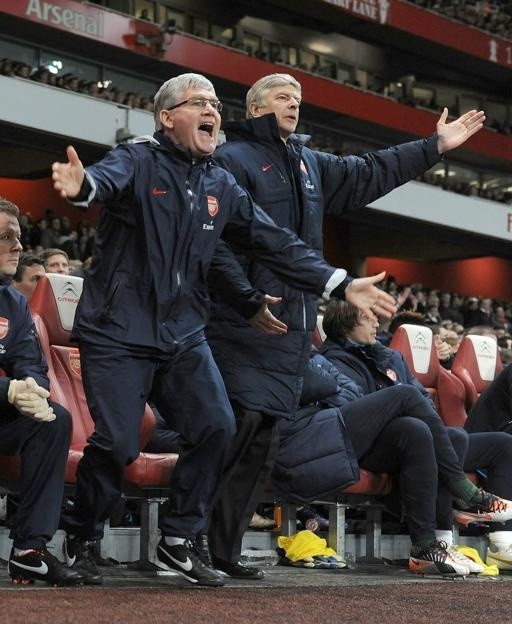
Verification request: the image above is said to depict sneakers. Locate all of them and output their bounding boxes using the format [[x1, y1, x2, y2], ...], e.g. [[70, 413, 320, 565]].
[[408, 543, 470, 578], [61, 536, 103, 586], [444, 543, 483, 574], [327, 554, 348, 568], [248, 513, 277, 530], [152, 538, 226, 586], [9, 545, 84, 586], [278, 546, 331, 569], [295, 507, 348, 531], [486, 543, 511, 571], [453, 490, 511, 527]]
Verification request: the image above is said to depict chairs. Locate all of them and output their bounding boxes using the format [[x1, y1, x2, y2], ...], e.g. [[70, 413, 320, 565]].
[[0, 315, 85, 488], [388, 324, 482, 488], [313, 315, 394, 568], [452, 334, 504, 413], [27, 272, 180, 572]]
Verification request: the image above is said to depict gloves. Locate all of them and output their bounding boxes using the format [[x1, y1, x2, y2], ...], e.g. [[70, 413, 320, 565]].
[[15, 376, 49, 418], [8, 379, 56, 423]]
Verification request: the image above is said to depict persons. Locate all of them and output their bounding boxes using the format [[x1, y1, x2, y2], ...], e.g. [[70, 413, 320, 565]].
[[0, 0, 512, 587]]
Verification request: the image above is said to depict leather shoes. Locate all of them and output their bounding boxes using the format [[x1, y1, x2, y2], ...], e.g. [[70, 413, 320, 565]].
[[187, 534, 213, 571], [212, 556, 264, 580]]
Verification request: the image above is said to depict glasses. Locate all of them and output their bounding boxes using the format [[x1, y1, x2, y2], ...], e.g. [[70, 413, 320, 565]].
[[167, 97, 223, 112]]
